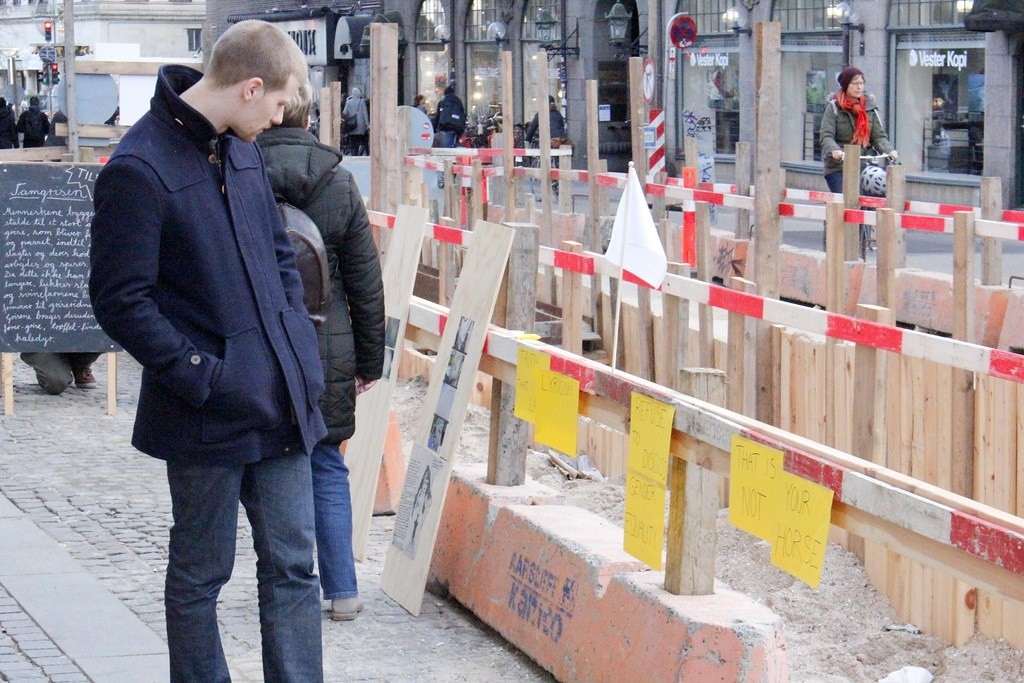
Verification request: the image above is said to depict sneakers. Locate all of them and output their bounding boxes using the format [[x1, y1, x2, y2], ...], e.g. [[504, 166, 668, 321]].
[[332, 596, 363, 621]]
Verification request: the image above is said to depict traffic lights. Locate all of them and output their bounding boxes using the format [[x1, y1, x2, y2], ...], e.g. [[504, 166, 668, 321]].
[[52, 62, 60, 84], [42, 63, 48, 85], [45, 21, 52, 42]]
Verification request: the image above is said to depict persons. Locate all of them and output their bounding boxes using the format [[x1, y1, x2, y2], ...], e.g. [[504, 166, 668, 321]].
[[44, 111, 67, 146], [104, 106, 120, 125], [252, 78, 386, 621], [819, 67, 898, 252], [412, 95, 426, 115], [0, 97, 19, 150], [432, 85, 466, 182], [20, 352, 102, 395], [525, 94, 565, 186], [89, 20, 327, 683], [343, 87, 370, 156], [17, 96, 50, 148]]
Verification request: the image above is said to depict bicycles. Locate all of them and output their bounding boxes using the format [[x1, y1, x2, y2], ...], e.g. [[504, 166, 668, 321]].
[[824, 152, 892, 264], [530, 137, 559, 203]]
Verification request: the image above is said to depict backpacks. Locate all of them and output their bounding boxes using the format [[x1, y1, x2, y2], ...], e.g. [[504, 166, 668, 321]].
[[271, 193, 328, 327], [439, 102, 465, 132], [24, 111, 44, 141]]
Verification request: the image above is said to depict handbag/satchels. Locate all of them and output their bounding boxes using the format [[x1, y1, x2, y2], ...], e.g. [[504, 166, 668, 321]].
[[345, 114, 357, 131]]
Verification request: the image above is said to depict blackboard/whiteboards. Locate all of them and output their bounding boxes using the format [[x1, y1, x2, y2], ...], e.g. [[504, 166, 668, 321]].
[[0, 161, 123, 352]]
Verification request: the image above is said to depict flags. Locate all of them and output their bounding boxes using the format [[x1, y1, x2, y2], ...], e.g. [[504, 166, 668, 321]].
[[605, 167, 668, 289]]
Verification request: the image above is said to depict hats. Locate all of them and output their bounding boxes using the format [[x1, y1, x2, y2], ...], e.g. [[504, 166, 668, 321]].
[[838, 67, 863, 93]]
